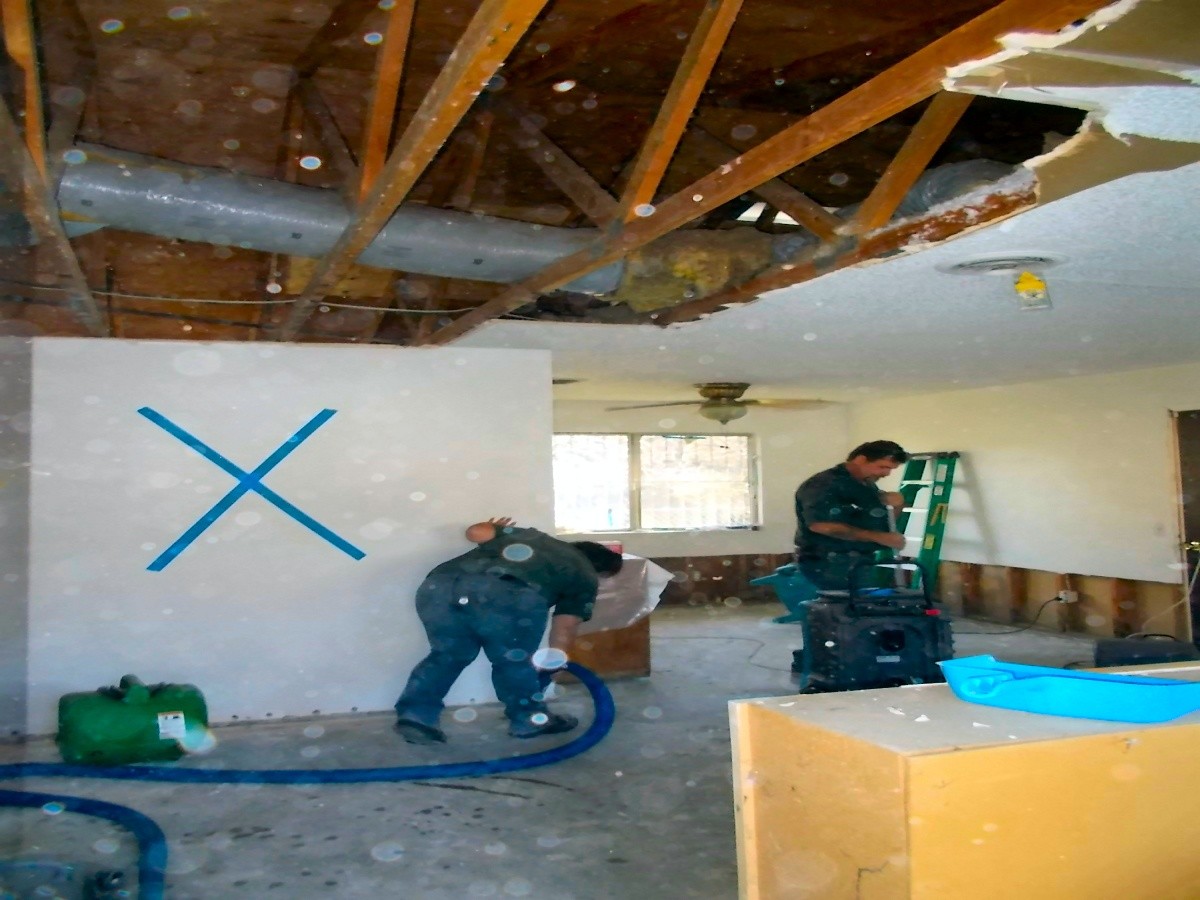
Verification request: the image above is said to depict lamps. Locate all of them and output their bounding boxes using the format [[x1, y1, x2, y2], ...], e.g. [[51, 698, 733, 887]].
[[699, 406, 746, 425]]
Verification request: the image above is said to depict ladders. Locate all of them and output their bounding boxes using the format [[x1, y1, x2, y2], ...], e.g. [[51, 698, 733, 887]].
[[878, 452, 960, 598]]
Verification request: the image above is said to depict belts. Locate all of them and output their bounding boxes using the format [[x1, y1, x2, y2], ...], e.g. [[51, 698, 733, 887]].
[[487, 572, 528, 587]]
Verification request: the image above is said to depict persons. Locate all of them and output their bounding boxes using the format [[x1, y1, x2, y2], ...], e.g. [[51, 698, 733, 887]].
[[789, 439, 915, 686], [391, 513, 624, 746]]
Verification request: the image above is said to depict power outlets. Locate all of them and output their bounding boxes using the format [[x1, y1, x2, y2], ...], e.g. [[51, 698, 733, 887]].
[[1058, 591, 1077, 602]]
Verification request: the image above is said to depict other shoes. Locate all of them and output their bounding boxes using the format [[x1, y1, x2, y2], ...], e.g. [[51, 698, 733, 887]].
[[393, 718, 447, 746], [509, 712, 578, 739]]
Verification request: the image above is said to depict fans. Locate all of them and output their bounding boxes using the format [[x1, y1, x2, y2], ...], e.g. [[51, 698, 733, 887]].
[[608, 382, 836, 414]]
